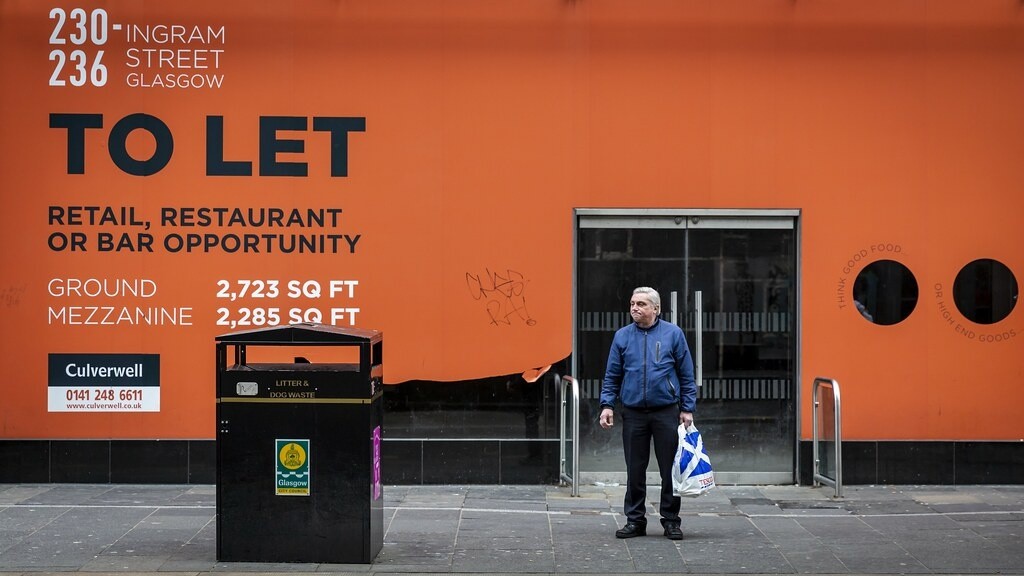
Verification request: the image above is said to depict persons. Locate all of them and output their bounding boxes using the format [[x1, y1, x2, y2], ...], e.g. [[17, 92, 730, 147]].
[[596, 286, 698, 540]]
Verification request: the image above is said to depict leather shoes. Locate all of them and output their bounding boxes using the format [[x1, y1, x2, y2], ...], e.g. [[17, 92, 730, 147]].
[[616, 524, 646, 538], [664, 522, 683, 540]]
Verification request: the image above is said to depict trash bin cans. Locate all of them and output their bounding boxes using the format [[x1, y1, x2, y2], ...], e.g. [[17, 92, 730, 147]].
[[213, 326, 383, 565]]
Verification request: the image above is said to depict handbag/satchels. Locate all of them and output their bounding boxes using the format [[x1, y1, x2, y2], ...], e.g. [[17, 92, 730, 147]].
[[671, 421, 717, 499]]
